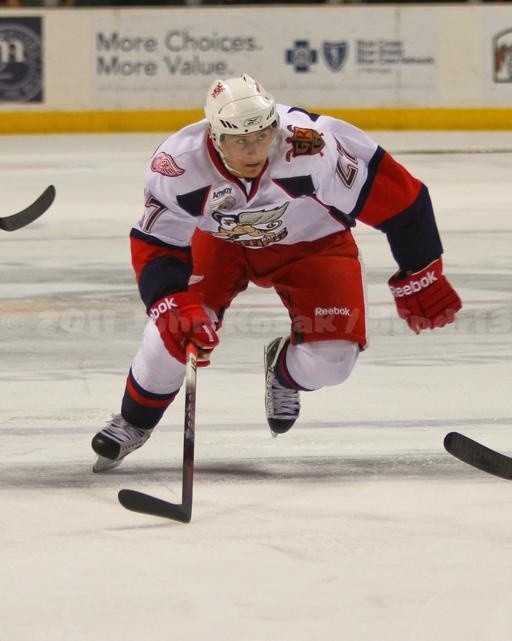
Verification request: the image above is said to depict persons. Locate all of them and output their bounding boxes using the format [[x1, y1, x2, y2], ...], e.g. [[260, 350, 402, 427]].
[[87, 69, 466, 464]]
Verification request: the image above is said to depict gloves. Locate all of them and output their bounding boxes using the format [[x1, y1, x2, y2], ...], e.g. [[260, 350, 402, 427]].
[[388, 256, 462, 335], [147, 291, 219, 367]]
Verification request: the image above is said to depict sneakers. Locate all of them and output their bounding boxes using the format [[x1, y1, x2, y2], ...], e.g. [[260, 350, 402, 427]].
[[91, 412, 153, 460], [265, 336, 300, 434]]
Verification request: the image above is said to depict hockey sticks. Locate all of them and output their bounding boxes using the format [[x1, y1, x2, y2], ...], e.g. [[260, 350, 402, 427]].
[[443, 432, 511, 481], [117, 340, 198, 524], [0, 185, 55, 231]]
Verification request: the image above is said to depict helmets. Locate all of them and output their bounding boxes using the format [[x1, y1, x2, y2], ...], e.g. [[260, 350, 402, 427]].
[[204, 73, 279, 150]]
